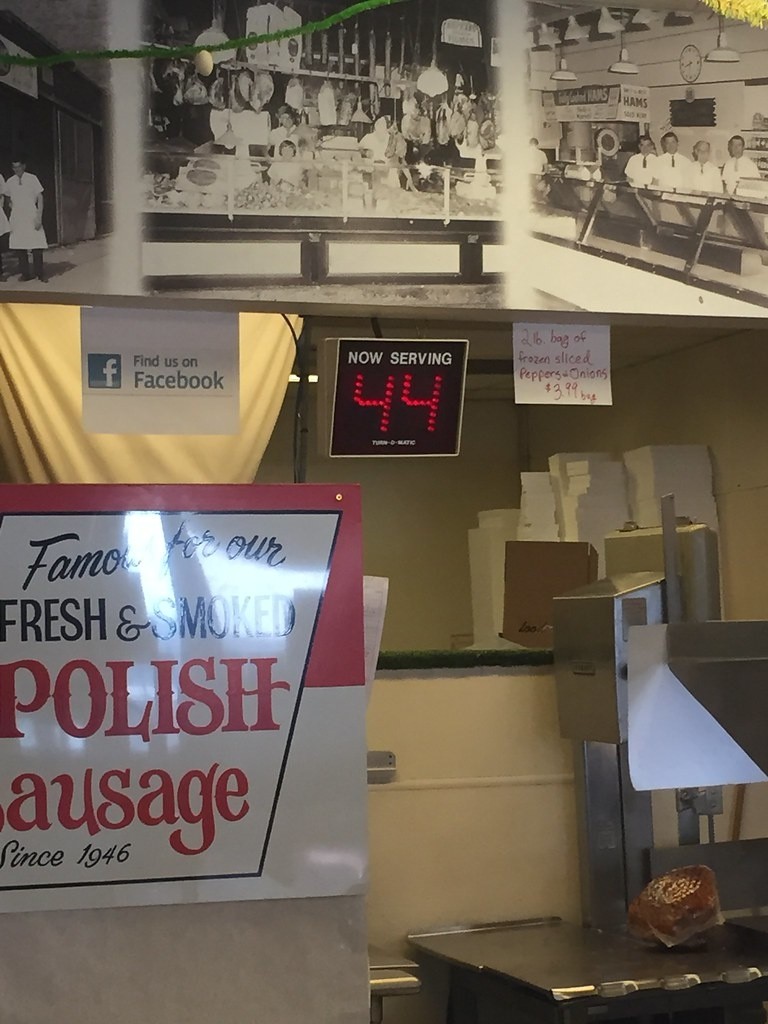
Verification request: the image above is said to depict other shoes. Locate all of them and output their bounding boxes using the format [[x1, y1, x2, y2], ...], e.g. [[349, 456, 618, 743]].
[[35, 272, 49, 282], [18, 274, 33, 281]]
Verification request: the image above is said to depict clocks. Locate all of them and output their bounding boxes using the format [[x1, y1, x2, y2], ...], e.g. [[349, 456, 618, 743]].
[[680, 44, 703, 83]]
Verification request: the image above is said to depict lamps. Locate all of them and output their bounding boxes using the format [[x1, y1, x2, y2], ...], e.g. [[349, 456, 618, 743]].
[[610, 6, 640, 74], [704, 14, 741, 62], [549, 4, 579, 81], [350, 95, 372, 124], [213, 64, 244, 150]]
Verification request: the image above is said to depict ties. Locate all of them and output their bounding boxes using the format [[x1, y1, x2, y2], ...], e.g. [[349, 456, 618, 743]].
[[734, 159, 738, 171], [672, 155, 675, 167], [642, 156, 647, 168], [700, 163, 703, 174]]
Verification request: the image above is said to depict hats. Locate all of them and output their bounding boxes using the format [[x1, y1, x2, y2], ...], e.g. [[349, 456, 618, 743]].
[[149, 50, 275, 114]]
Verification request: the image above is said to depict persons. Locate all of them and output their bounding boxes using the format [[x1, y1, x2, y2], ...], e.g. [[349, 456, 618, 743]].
[[624, 132, 760, 196], [266, 87, 552, 205], [0, 157, 49, 283]]
